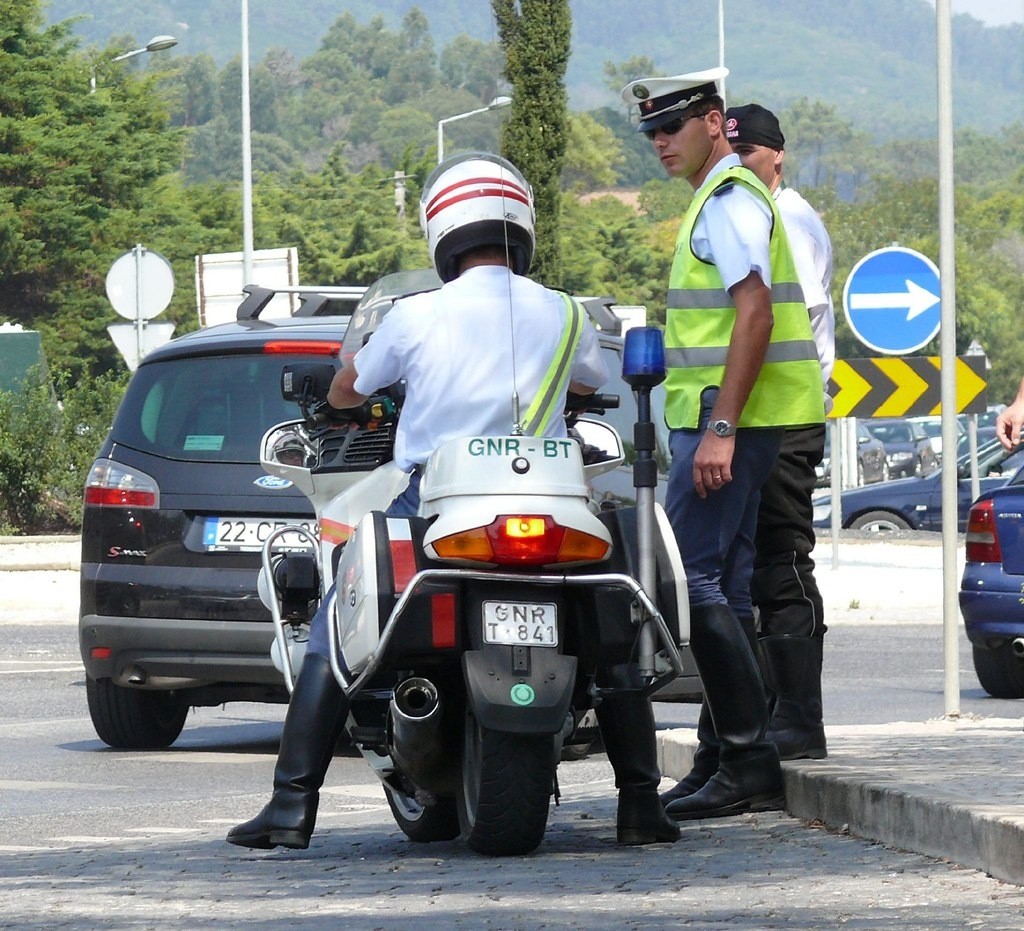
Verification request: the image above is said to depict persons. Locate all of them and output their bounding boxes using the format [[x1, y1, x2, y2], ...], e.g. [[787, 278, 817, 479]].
[[223, 152, 682, 848], [996, 377, 1024, 451], [622, 65, 836, 822]]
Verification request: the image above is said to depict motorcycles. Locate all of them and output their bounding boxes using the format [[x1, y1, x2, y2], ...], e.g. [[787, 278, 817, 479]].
[[282, 269, 693, 858]]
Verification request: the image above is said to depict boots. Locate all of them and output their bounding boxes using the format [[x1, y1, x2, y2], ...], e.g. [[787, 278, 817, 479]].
[[666, 604, 785, 819], [226, 652, 350, 848], [659, 615, 759, 808], [591, 663, 681, 845], [757, 632, 827, 761]]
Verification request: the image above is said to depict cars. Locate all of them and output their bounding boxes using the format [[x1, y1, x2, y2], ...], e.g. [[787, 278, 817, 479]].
[[812, 401, 1023, 534], [958, 462, 1024, 699]]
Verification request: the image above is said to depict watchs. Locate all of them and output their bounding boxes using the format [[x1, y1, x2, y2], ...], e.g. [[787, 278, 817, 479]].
[[707, 419, 736, 437]]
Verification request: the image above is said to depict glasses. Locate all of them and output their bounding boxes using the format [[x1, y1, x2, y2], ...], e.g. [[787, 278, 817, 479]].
[[643, 112, 711, 141]]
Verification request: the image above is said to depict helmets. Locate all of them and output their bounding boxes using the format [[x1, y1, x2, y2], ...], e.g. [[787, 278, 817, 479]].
[[419, 152, 535, 285]]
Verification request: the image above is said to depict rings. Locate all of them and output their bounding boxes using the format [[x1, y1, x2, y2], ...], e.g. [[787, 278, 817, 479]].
[[713, 475, 720, 479]]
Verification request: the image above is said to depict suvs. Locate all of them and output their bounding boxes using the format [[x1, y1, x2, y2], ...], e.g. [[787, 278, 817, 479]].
[[78, 279, 763, 763]]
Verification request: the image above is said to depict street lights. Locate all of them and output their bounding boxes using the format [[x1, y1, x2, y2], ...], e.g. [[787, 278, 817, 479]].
[[437, 94, 514, 166], [89, 35, 178, 96]]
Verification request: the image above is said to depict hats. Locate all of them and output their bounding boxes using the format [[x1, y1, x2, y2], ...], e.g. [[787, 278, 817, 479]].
[[622, 67, 729, 133], [725, 104, 785, 151]]
